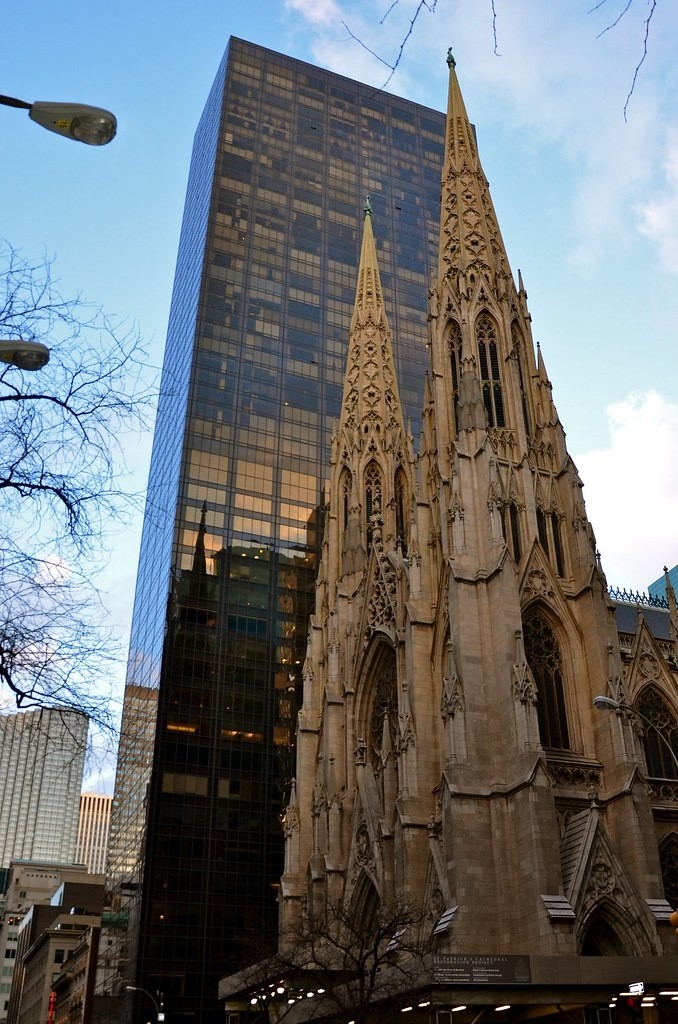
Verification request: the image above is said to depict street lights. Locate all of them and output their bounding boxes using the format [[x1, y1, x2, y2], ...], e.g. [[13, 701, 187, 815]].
[[125, 985, 165, 1024]]
